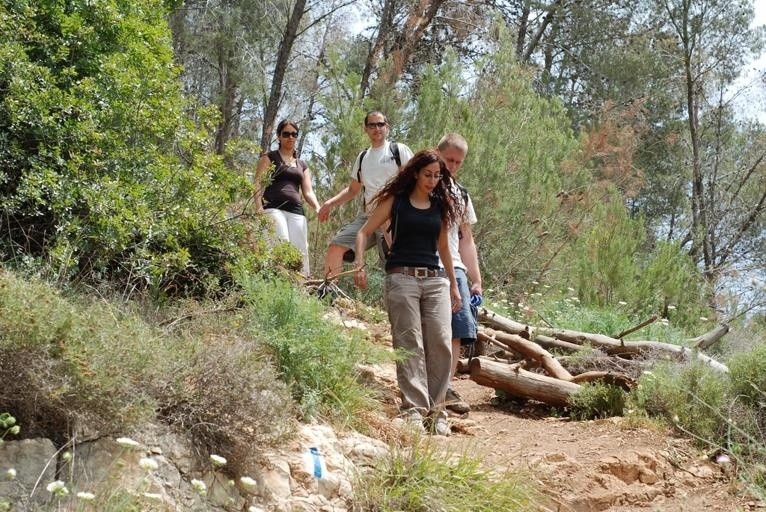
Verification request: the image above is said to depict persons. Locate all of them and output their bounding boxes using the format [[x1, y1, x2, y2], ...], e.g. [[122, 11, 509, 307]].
[[379, 132, 482, 411], [254, 119, 328, 275], [319, 111, 415, 278], [353, 149, 462, 437]]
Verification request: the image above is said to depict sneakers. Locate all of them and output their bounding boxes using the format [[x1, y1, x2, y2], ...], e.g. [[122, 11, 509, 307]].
[[406, 388, 472, 436]]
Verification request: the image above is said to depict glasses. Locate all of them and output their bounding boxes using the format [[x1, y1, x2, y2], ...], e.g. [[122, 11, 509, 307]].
[[368, 122, 385, 128], [282, 131, 298, 138]]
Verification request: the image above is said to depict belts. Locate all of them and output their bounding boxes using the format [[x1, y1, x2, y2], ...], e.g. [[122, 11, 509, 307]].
[[385, 266, 447, 278]]
